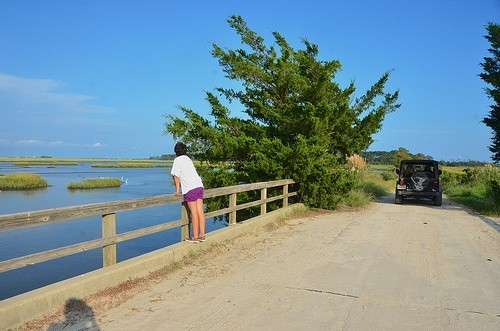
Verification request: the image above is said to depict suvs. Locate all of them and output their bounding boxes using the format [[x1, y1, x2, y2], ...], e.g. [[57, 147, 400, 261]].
[[395, 159, 443, 206]]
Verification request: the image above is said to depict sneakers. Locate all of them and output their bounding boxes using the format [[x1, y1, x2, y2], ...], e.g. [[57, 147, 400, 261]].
[[185, 236, 205, 243]]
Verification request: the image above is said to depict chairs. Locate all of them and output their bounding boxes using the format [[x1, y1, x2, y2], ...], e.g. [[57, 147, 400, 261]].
[[403, 166, 417, 177], [423, 166, 433, 174]]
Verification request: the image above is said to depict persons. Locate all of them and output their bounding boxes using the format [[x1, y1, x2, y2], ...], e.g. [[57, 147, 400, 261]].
[[170, 142, 206, 244]]
